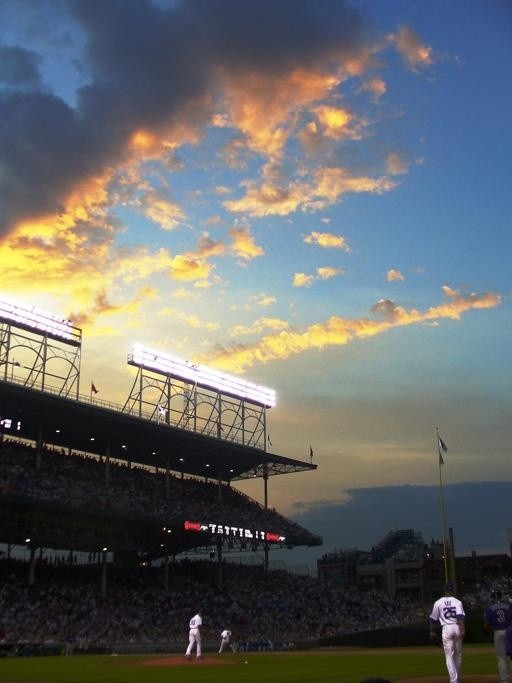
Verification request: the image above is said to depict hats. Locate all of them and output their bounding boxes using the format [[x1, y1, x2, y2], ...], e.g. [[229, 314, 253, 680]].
[[444, 582, 454, 592], [492, 590, 502, 599]]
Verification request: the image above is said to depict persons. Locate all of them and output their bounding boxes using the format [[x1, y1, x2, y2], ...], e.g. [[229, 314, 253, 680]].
[[428, 584, 469, 683], [184, 609, 204, 659], [455, 578, 510, 610], [2, 437, 318, 549], [483, 588, 511, 682], [3, 551, 434, 654]]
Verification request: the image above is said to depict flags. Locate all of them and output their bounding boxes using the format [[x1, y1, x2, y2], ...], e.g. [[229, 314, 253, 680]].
[[14, 360, 20, 368], [91, 383, 99, 394], [158, 404, 165, 415], [438, 436, 448, 465]]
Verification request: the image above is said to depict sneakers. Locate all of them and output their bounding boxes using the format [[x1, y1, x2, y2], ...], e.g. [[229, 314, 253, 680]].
[[185, 653, 237, 660]]
